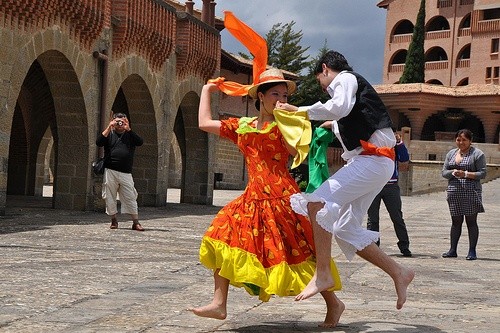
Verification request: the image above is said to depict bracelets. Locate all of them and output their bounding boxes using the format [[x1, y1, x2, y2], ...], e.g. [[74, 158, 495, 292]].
[[465, 170, 468, 179]]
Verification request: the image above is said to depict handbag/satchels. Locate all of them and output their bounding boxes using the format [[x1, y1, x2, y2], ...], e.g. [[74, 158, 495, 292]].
[[92, 158, 104, 176]]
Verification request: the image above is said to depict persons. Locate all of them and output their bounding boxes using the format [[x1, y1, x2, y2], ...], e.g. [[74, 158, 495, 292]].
[[96, 113, 144, 231], [442, 129, 487, 260], [187, 69, 345, 328], [275, 50, 415, 310], [366, 133, 413, 257]]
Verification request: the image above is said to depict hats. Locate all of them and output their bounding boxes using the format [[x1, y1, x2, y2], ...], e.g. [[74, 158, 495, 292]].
[[247, 69, 296, 99]]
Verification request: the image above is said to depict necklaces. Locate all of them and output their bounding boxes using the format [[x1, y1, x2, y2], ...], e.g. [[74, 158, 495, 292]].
[[112, 129, 125, 142]]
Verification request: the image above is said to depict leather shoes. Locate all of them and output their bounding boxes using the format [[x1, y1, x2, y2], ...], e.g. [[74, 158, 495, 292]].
[[132, 223, 145, 231], [110, 222, 118, 229]]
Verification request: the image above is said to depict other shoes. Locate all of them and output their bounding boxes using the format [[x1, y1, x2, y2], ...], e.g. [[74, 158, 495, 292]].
[[401, 249, 411, 256], [442, 249, 457, 257], [466, 251, 476, 260]]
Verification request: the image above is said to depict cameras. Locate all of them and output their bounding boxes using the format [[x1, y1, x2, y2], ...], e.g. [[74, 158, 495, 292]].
[[114, 121, 123, 126]]
